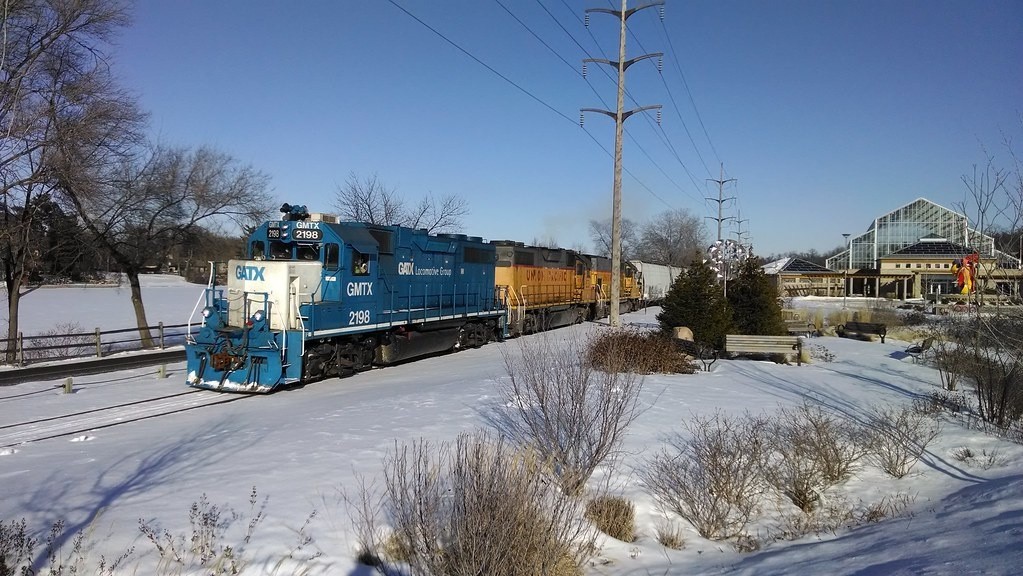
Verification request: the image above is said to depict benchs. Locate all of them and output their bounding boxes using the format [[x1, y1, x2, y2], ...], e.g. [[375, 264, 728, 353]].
[[904, 336, 934, 363], [721, 334, 804, 367], [785, 319, 818, 338], [835, 321, 887, 343]]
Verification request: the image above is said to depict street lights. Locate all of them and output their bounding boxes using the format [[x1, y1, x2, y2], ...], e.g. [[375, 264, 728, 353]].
[[842, 234, 851, 310]]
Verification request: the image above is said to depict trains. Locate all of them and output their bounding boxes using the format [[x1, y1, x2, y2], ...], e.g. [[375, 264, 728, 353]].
[[181, 202, 689, 393]]
